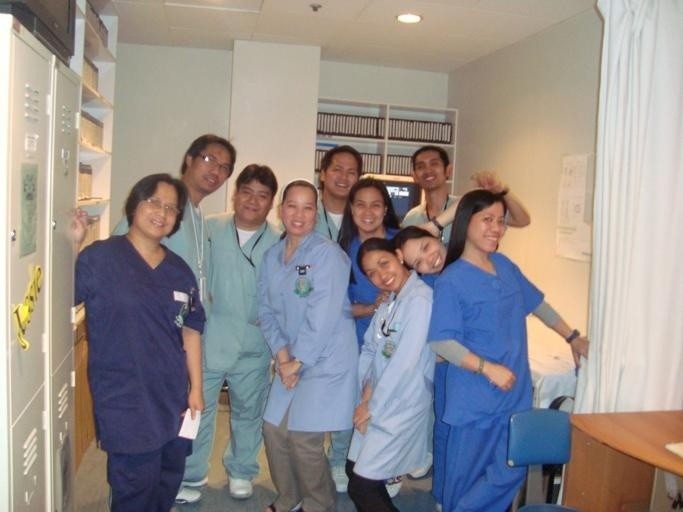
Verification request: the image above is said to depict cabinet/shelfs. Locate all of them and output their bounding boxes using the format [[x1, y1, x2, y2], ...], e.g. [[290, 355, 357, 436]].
[[0, 13, 81, 512], [73, 317, 96, 475], [77, 17, 117, 206], [315, 96, 458, 196]]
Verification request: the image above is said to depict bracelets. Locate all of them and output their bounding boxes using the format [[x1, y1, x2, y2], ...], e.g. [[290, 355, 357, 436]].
[[568, 330, 579, 343], [476, 359, 484, 375], [502, 189, 509, 195]]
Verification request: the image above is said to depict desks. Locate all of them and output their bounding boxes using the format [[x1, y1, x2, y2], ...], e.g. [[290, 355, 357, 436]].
[[561, 410, 683, 512]]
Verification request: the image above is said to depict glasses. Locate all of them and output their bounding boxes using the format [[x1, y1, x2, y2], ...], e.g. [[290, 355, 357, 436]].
[[145, 197, 180, 214], [195, 155, 231, 175]]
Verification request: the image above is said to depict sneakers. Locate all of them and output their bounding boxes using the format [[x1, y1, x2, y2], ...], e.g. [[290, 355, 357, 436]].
[[410, 452, 433, 478], [331, 465, 349, 492], [229, 472, 253, 498], [176, 475, 209, 503]]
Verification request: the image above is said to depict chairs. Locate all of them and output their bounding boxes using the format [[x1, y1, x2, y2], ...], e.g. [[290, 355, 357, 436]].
[[508, 409, 582, 512]]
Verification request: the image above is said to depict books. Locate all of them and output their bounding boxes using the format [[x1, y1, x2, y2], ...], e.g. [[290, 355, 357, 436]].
[[316, 111, 453, 177]]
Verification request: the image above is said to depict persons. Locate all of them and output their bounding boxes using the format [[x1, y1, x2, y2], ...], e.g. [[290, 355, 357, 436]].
[[401, 146, 531, 476], [338, 180, 465, 496], [394, 225, 450, 289], [313, 145, 363, 490], [345, 238, 435, 512], [115, 135, 235, 505], [259, 180, 361, 512], [73, 174, 206, 512], [182, 164, 279, 499], [423, 189, 592, 511]]
